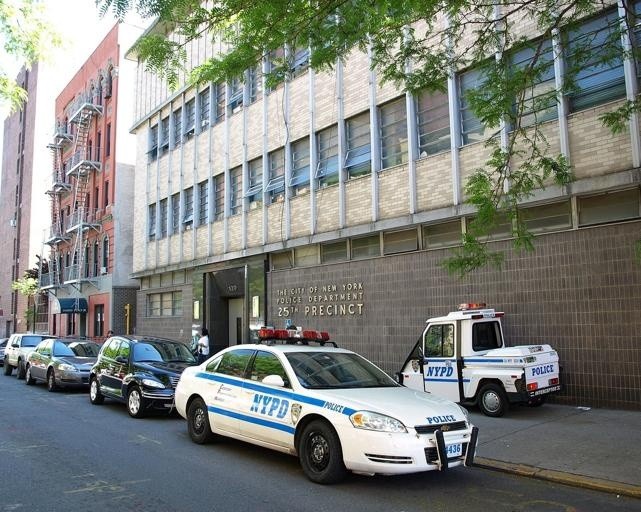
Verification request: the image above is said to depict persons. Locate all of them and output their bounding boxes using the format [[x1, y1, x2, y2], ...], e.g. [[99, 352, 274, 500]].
[[103, 329, 114, 343], [195, 328, 210, 364]]
